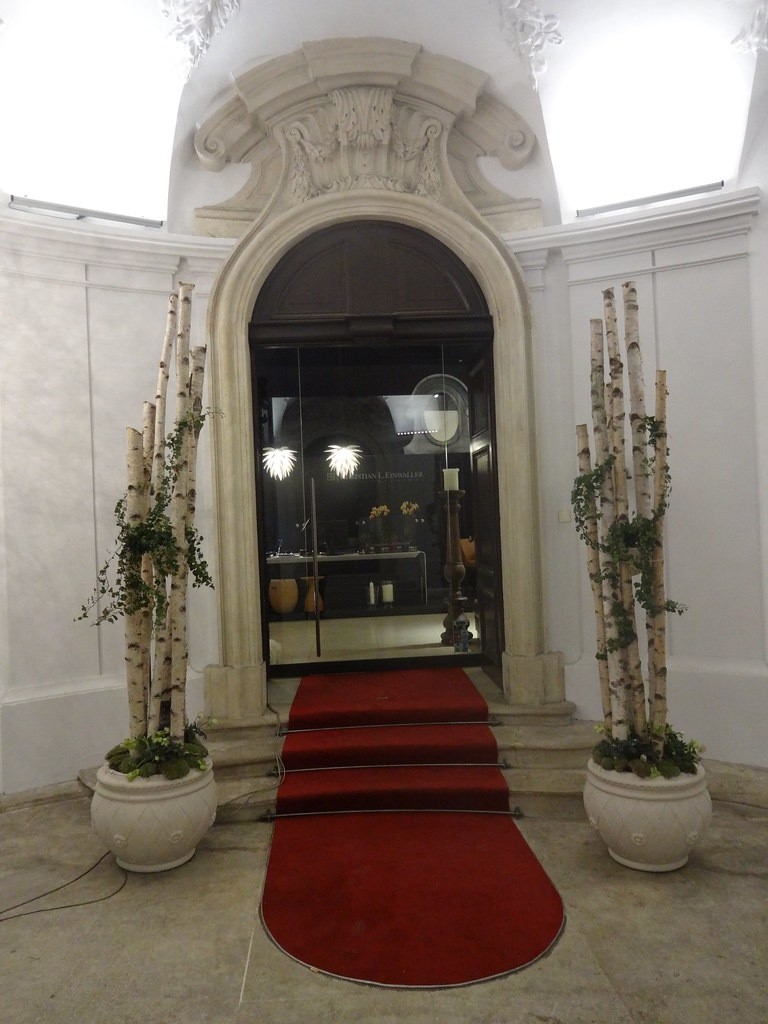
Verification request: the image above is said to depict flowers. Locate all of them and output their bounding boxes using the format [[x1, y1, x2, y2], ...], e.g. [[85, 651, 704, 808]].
[[401, 501, 420, 540], [368, 504, 391, 543]]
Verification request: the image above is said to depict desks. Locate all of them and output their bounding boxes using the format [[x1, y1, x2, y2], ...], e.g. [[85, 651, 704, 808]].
[[267, 550, 428, 607]]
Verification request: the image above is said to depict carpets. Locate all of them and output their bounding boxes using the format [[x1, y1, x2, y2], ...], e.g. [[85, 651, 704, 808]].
[[259, 664, 569, 992]]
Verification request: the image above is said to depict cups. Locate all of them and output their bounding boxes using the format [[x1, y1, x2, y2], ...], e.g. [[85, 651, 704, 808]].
[[269, 579, 297, 614]]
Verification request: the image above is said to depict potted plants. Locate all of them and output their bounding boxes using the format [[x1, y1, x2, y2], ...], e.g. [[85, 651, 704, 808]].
[[567, 277, 715, 874], [72, 280, 218, 873]]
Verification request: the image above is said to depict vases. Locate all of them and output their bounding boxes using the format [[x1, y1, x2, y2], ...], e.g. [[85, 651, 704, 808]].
[[302, 575, 327, 612], [269, 579, 299, 615], [396, 516, 418, 552], [373, 517, 390, 552]]
